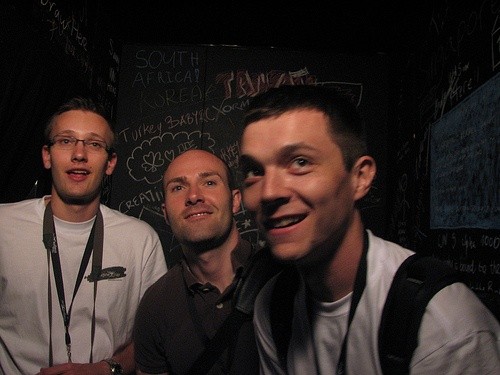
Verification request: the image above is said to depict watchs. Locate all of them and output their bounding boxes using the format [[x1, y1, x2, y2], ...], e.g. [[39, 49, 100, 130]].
[[101, 358, 124, 375]]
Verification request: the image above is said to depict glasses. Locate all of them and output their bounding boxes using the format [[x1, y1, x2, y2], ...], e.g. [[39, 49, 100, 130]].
[[48, 134, 115, 154]]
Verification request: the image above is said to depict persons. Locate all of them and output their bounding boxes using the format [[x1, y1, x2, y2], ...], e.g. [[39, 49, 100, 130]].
[[238, 83, 499, 375], [132, 147, 283, 375], [0, 98, 168, 375]]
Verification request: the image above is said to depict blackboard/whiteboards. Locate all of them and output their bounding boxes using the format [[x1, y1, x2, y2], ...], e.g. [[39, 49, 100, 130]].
[[0, 0, 500, 320]]
